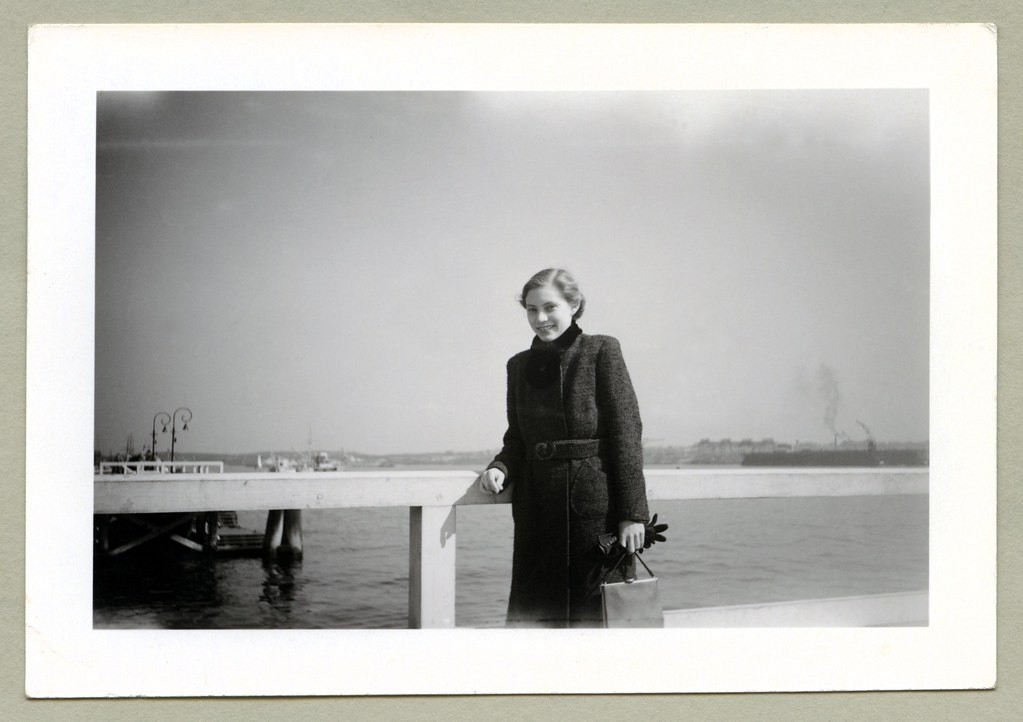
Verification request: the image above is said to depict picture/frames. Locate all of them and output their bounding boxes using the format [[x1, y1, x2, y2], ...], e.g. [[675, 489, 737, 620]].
[[24, 21, 1001, 701]]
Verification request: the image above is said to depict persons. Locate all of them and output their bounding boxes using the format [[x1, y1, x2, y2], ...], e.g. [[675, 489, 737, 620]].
[[480, 270, 650, 629], [113, 454, 123, 473], [140, 449, 163, 472]]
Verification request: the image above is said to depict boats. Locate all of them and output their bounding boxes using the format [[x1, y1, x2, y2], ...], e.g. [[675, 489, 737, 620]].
[[252, 450, 341, 473]]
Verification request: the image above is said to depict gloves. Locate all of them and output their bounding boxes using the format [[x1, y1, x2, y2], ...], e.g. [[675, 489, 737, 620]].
[[597, 513, 668, 560]]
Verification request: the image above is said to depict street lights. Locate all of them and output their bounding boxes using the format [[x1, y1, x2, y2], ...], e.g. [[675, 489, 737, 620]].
[[152, 411, 172, 470], [171, 407, 192, 471]]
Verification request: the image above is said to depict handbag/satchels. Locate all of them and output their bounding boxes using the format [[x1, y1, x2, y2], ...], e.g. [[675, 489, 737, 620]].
[[600, 552, 665, 628]]
